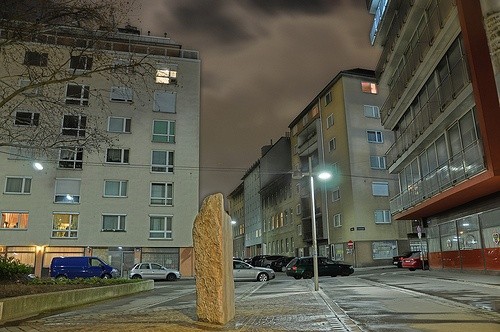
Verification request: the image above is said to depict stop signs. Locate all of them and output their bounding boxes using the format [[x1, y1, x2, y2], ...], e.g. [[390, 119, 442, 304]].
[[347, 240, 353, 248]]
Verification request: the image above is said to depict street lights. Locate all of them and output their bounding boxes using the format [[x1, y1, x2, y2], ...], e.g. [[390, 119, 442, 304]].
[[291, 157, 331, 292]]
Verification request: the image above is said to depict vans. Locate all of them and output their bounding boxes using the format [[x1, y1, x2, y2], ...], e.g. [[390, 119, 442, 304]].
[[49, 256, 120, 280]]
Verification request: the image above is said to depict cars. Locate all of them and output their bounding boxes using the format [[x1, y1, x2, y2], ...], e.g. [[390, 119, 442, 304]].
[[249, 254, 296, 273], [401, 253, 423, 271], [286, 256, 354, 279], [232, 259, 275, 282], [129, 262, 181, 282], [390, 250, 421, 269]]
